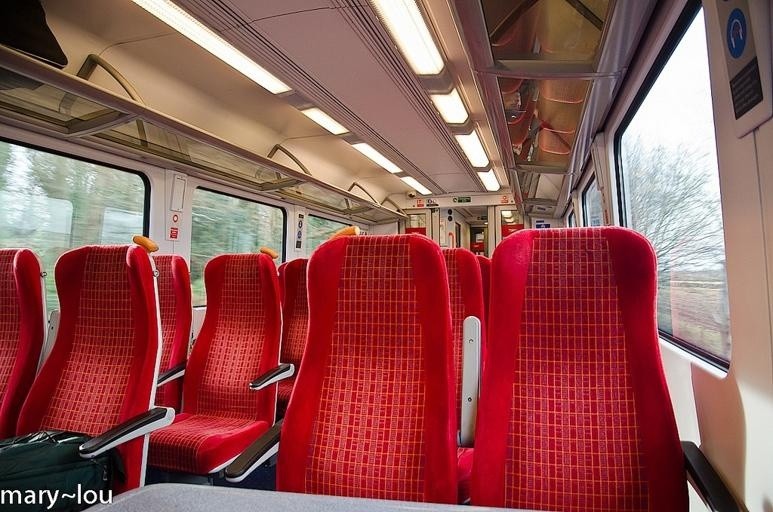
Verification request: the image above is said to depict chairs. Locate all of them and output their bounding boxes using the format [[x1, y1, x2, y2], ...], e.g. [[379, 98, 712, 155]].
[[0, 225, 754, 512]]
